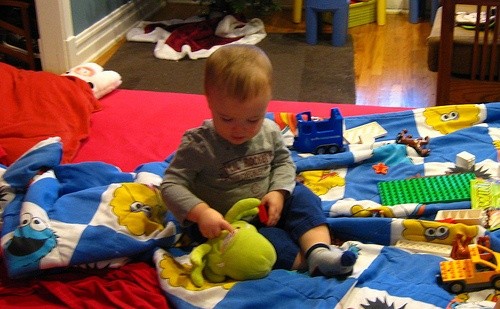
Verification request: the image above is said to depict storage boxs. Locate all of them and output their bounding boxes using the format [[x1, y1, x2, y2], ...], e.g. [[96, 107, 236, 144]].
[[435, 209, 485, 226], [456, 150, 475, 171], [318, 0, 377, 29]]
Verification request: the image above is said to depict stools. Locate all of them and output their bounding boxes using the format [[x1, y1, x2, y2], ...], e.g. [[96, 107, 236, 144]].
[[0, 0, 39, 70]]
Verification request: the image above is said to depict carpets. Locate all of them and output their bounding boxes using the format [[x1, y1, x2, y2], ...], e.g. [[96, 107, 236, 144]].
[[71, 88, 419, 171]]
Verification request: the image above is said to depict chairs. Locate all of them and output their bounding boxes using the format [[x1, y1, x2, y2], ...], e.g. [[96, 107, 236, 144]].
[[426, 4, 500, 78], [435, 0, 500, 105]]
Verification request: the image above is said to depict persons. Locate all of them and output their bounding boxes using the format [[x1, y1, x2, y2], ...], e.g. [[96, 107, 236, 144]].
[[159, 45, 357, 274]]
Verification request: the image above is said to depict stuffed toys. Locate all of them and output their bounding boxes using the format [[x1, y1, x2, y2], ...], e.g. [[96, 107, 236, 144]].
[[189, 199, 277, 287]]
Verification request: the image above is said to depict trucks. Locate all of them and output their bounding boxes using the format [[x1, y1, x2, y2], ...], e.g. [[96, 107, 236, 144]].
[[296, 107, 345, 154], [440, 244, 500, 292]]
